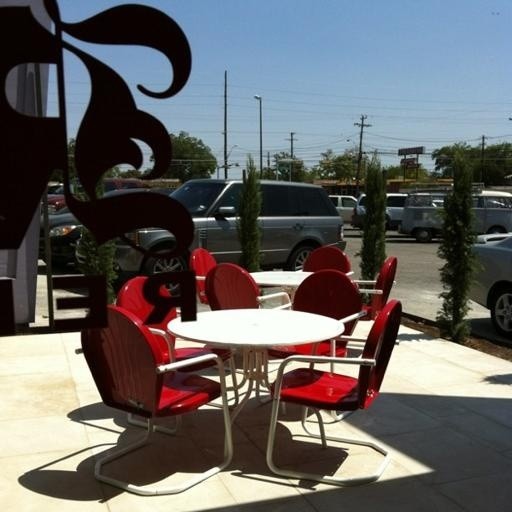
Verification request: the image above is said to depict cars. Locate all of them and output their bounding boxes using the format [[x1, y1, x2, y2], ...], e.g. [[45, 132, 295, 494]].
[[467, 230, 512, 339]]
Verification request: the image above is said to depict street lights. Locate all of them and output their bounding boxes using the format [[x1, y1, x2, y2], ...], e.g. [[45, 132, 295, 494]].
[[253, 94, 263, 179], [217, 164, 240, 180], [224, 144, 239, 179], [346, 138, 362, 198]]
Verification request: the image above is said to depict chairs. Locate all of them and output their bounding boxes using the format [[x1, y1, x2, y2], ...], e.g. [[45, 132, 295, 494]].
[[199, 262, 293, 375], [74, 306, 235, 497], [191, 247, 397, 321], [264, 299, 402, 487], [255, 268, 364, 421], [115, 276, 240, 434]]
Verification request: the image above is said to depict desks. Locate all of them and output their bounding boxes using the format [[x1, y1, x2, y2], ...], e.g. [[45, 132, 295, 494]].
[[166, 308, 345, 430]]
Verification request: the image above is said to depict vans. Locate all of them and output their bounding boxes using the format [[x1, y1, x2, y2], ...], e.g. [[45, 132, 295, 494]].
[[323, 189, 512, 245]]
